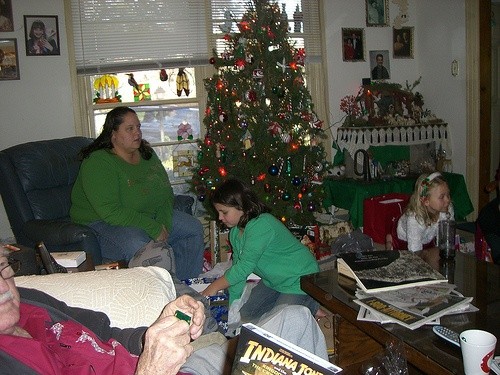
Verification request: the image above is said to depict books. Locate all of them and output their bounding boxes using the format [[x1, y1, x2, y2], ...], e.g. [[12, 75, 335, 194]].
[[231, 322, 343, 375], [336, 250, 480, 331]]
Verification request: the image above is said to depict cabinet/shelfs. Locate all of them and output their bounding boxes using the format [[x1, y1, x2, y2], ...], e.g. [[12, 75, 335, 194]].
[[320, 121, 474, 242], [92, 97, 208, 224]]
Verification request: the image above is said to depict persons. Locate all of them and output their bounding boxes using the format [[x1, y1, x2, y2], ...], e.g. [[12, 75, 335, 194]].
[[391, 172, 455, 270], [367, 0, 379, 23], [344, 33, 362, 59], [199, 178, 328, 321], [0, 241, 330, 375], [372, 54, 389, 79], [27, 21, 57, 54], [394, 30, 410, 55], [69, 106, 204, 280]]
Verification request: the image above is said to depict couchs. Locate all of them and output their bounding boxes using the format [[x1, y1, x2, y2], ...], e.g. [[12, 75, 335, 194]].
[[10, 266, 176, 334]]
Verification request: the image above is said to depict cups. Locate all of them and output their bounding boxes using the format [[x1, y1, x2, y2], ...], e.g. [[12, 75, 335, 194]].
[[460, 329, 497, 375], [385, 336, 408, 375], [438, 220, 456, 259]]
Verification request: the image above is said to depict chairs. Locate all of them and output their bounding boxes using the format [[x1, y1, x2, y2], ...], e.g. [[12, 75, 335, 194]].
[[0, 136, 196, 285]]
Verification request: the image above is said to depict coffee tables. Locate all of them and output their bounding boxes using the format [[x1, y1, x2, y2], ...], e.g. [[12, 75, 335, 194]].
[[300, 245, 500, 375]]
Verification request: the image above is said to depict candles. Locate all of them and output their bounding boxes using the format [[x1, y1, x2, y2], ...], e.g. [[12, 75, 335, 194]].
[[106, 84, 109, 98], [112, 84, 115, 98], [100, 86, 104, 98]]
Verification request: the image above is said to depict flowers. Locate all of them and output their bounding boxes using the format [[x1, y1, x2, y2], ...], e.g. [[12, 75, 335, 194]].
[[176, 122, 194, 140]]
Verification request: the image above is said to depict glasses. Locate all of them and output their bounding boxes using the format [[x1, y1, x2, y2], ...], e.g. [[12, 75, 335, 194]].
[[0, 255, 21, 280]]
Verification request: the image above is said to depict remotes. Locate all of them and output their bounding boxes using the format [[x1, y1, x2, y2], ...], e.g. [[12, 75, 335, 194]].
[[432, 325, 461, 348]]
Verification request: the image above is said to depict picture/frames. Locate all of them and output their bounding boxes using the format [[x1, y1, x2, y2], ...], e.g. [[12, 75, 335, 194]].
[[365, 0, 390, 27], [0, 0, 14, 32], [23, 15, 60, 56], [392, 26, 414, 60], [173, 150, 193, 177], [342, 27, 367, 63], [0, 38, 20, 81]]
